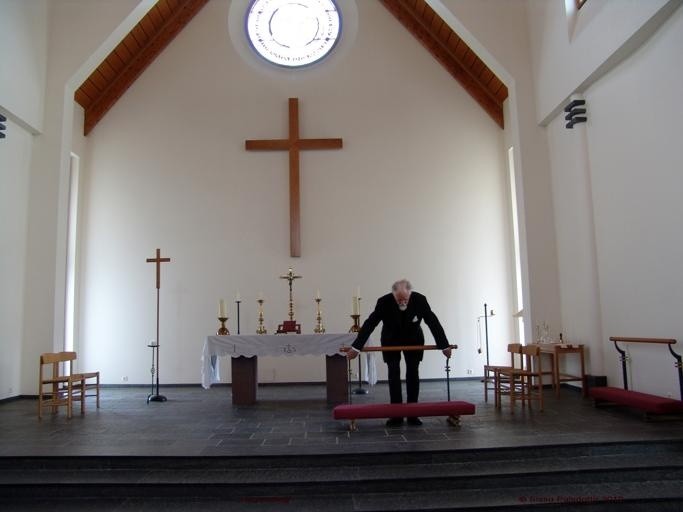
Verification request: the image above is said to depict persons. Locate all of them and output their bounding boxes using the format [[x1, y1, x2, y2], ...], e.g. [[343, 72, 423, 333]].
[[346, 279, 451, 426]]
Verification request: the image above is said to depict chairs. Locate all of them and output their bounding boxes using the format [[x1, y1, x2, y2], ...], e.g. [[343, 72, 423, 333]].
[[483, 343, 542, 413], [37, 351, 99, 418]]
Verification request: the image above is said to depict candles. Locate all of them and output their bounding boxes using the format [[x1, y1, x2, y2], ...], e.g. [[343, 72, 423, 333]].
[[217, 283, 361, 322]]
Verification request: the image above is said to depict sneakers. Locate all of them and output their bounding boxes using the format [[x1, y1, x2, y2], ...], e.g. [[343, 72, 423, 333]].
[[407, 417, 422, 426], [386, 417, 402, 427]]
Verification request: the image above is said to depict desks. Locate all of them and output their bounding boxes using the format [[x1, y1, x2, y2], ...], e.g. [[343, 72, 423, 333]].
[[533, 343, 586, 399]]
